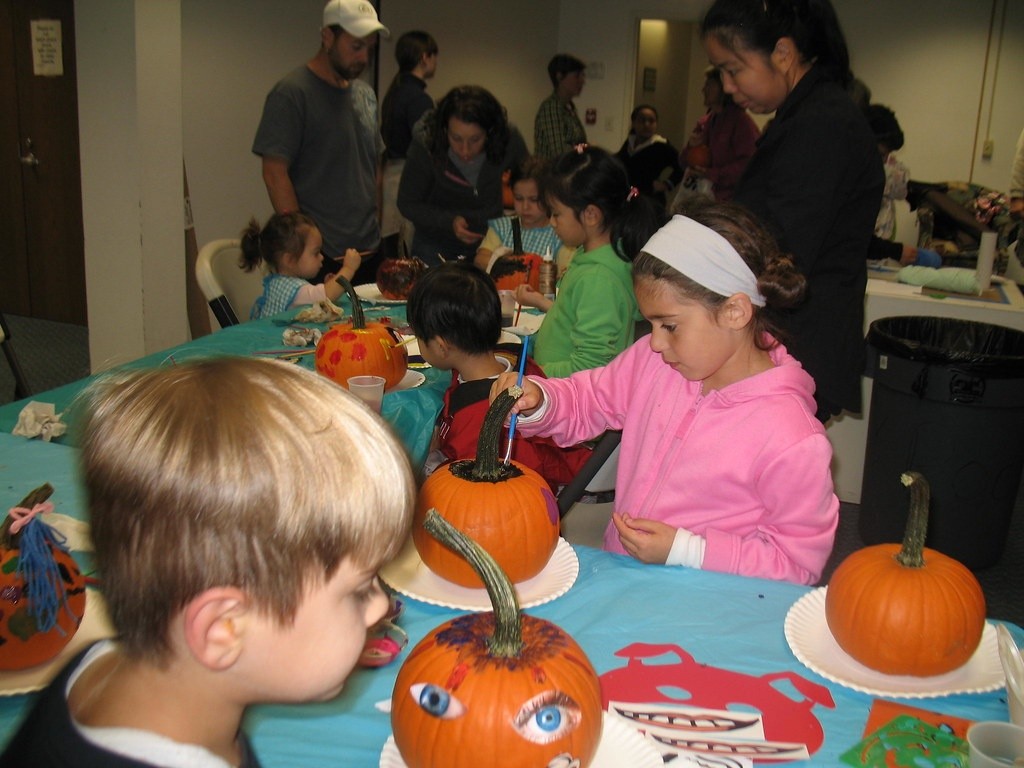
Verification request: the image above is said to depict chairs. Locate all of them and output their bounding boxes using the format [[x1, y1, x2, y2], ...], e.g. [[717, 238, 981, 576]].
[[195, 237, 265, 328], [558, 430, 624, 548]]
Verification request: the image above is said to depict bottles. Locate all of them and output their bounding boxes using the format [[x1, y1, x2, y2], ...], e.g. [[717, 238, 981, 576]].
[[538, 246, 557, 312]]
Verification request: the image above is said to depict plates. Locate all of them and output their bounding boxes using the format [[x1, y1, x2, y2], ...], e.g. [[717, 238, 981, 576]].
[[383, 369, 426, 394], [497, 330, 521, 343], [783, 585, 1006, 699], [0, 587, 121, 698], [400, 334, 422, 356], [346, 282, 407, 304], [375, 531, 580, 613], [379, 710, 664, 768]]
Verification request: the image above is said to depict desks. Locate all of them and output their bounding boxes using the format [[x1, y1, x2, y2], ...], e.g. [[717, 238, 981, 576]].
[[1, 289, 1023, 768]]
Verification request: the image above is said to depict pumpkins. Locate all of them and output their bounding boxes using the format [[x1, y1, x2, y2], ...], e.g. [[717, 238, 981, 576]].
[[825, 471, 986, 678], [374, 223, 423, 300], [411, 381, 560, 588], [315, 275, 409, 391], [489, 216, 546, 289], [390, 509, 602, 768], [1, 482, 86, 672]]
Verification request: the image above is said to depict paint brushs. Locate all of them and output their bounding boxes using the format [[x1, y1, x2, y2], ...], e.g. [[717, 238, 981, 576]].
[[503, 336, 529, 466], [288, 324, 325, 335], [437, 254, 447, 263], [333, 251, 374, 261], [387, 335, 416, 348], [254, 348, 317, 358], [515, 260, 534, 327]]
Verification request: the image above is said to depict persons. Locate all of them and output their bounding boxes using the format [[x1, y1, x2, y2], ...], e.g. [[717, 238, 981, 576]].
[[1009, 130, 1024, 222], [406, 262, 594, 496], [238, 208, 361, 320], [0, 342, 418, 768], [487, 202, 840, 584], [868, 104, 919, 265], [535, 55, 588, 180], [251, 0, 391, 286], [614, 105, 680, 226], [510, 144, 646, 380], [699, 0, 888, 422], [378, 30, 438, 260], [473, 158, 579, 279], [396, 84, 530, 259], [682, 67, 762, 198]]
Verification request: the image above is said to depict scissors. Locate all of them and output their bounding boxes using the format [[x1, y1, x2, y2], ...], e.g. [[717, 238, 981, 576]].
[[271, 318, 298, 328]]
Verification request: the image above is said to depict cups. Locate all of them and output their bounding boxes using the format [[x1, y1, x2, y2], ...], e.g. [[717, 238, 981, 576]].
[[966, 721, 1024, 768], [497, 289, 516, 329], [347, 375, 387, 416]]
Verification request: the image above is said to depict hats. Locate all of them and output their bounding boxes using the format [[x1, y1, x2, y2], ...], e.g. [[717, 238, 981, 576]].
[[320, 1, 391, 38]]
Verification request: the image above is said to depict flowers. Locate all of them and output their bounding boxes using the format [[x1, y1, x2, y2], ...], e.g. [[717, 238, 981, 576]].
[[973, 191, 1007, 221]]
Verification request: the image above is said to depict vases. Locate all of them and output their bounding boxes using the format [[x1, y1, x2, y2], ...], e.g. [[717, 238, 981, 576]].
[[974, 228, 997, 297]]
